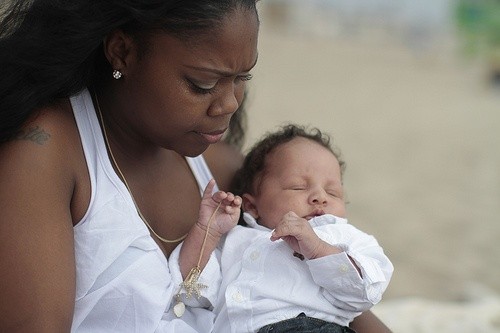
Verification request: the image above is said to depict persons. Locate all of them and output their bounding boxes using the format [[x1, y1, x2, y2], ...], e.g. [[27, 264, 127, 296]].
[[167, 125, 394, 333], [0, 0, 389, 333]]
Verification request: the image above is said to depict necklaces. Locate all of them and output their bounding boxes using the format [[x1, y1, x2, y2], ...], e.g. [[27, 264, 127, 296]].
[[94, 89, 221, 318]]
